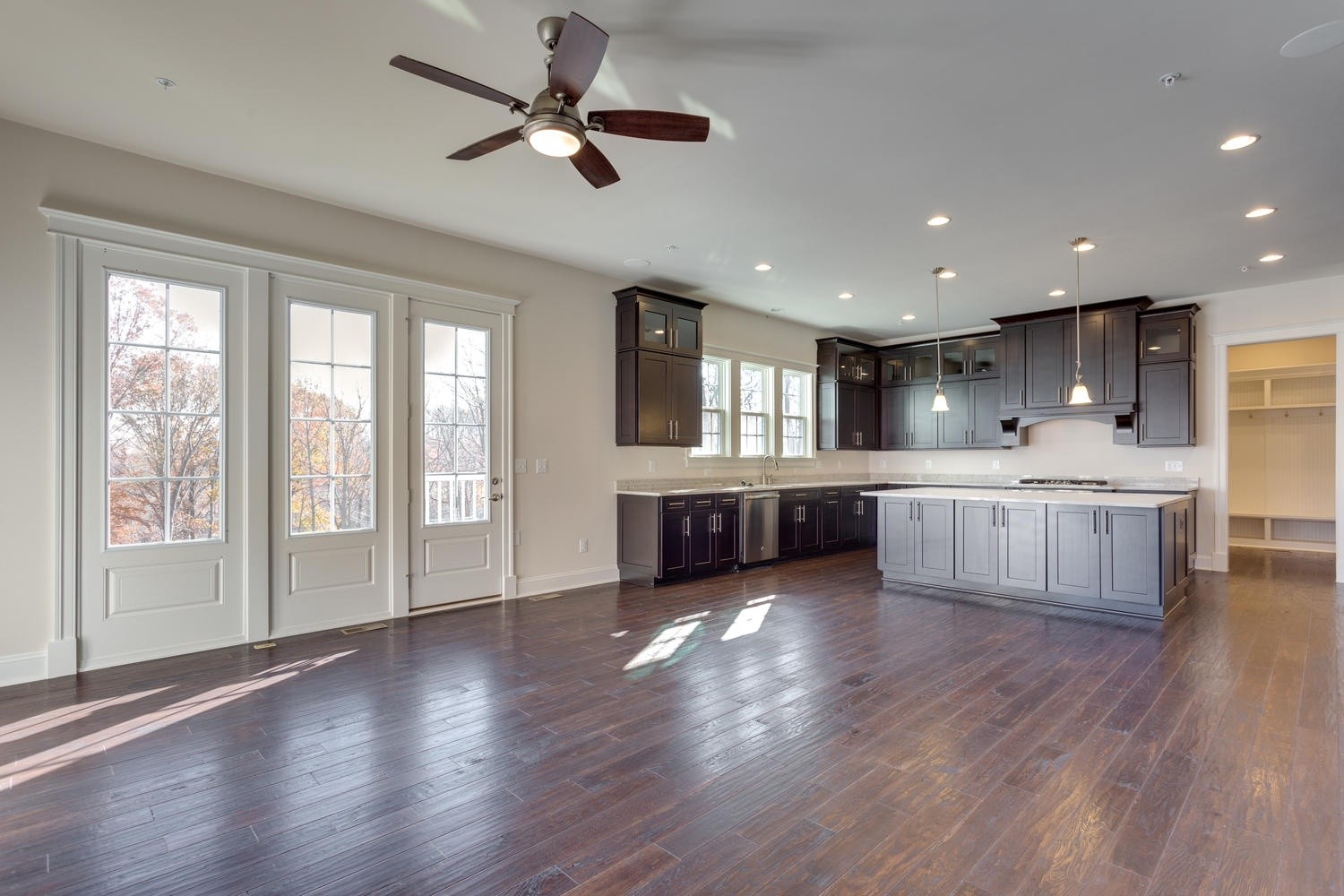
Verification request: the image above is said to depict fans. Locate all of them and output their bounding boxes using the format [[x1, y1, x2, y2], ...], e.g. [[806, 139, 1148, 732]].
[[385, 10, 713, 191]]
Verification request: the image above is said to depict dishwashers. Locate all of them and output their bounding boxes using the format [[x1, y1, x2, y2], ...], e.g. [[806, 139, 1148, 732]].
[[738, 492, 779, 570]]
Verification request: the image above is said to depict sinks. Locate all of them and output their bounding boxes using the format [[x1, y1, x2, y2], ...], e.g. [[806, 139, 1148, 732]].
[[720, 480, 794, 491]]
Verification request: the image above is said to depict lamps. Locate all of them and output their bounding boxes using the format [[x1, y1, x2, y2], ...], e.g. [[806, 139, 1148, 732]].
[[928, 267, 953, 411], [1068, 237, 1093, 406]]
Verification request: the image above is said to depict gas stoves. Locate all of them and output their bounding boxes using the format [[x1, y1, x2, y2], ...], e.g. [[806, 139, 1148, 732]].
[[1018, 479, 1108, 486]]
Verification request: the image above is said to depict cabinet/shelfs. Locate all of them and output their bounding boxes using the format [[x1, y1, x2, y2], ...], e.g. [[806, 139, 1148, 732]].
[[875, 496, 1190, 620], [878, 344, 939, 388], [618, 484, 882, 588], [937, 336, 1002, 382], [998, 322, 1025, 417], [1025, 312, 1104, 416], [1104, 308, 1138, 410], [817, 339, 879, 387], [1137, 316, 1197, 365], [817, 381, 878, 453], [614, 347, 704, 449], [611, 286, 709, 359], [938, 379, 1009, 451], [1137, 361, 1197, 448], [877, 384, 938, 451]]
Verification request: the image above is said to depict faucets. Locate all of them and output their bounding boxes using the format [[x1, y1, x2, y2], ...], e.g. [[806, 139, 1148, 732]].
[[762, 455, 779, 485]]
